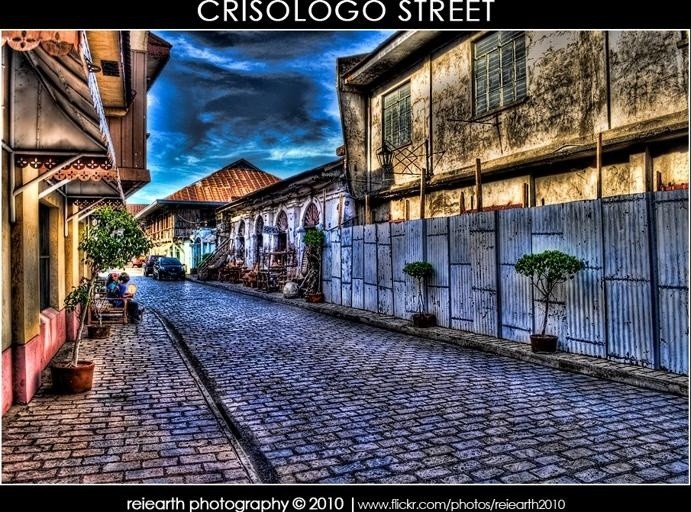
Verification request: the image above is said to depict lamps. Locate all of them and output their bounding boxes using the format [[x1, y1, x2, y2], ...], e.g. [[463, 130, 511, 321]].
[[378, 133, 430, 176]]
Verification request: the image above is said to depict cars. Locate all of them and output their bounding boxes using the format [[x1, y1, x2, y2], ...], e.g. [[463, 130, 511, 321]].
[[152, 257, 186, 281], [131, 254, 147, 269]]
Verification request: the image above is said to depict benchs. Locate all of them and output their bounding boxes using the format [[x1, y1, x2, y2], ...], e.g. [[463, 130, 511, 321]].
[[86, 284, 128, 327]]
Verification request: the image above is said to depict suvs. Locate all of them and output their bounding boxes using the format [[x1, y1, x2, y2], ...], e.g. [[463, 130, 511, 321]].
[[142, 255, 165, 277]]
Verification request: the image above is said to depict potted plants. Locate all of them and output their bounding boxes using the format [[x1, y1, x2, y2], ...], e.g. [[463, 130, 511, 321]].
[[50, 195, 156, 396], [300, 226, 328, 303], [515, 248, 588, 355], [87, 295, 115, 339], [400, 261, 442, 329]]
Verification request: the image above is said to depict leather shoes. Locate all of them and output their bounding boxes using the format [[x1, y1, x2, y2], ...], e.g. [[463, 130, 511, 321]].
[[129, 318, 139, 324], [138, 307, 145, 315]]
